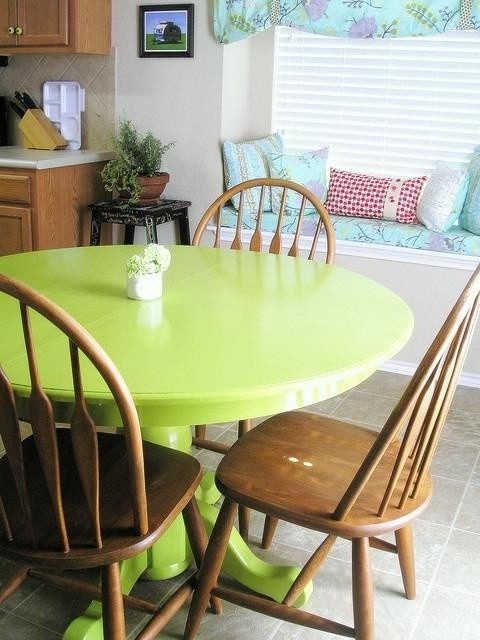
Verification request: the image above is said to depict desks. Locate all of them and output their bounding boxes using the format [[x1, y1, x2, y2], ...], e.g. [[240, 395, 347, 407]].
[[0, 245, 415, 640]]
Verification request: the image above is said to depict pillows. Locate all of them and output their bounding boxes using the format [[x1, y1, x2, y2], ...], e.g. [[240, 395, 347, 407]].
[[266, 146, 333, 215], [417, 162, 470, 233], [458, 142, 480, 236], [223, 129, 282, 212], [323, 166, 427, 223]]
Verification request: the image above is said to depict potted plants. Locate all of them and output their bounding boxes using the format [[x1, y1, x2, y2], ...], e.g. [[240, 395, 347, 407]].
[[97, 119, 175, 206]]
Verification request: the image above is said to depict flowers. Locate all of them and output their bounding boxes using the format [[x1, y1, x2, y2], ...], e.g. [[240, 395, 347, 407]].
[[124, 242, 171, 280]]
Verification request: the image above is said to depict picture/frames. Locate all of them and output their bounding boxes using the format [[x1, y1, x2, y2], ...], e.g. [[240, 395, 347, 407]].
[[137, 3, 195, 58]]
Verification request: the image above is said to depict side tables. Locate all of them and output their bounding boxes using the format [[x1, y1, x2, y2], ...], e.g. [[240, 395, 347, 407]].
[[87, 198, 192, 245]]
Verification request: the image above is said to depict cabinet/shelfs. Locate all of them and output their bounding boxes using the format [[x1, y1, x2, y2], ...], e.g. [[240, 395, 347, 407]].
[[0, 162, 112, 257], [0, 0, 112, 56]]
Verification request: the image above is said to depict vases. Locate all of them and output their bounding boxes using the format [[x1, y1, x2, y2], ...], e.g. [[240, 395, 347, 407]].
[[126, 274, 163, 301]]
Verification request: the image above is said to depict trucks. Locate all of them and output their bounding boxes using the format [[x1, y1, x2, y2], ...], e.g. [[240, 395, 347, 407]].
[[153, 21, 182, 46]]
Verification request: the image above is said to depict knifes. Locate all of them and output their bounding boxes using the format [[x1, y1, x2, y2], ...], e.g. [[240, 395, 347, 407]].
[[8, 90, 36, 117]]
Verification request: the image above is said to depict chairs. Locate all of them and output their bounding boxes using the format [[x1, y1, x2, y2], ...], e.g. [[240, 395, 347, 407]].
[[185, 263, 480, 640], [0, 274, 222, 640], [192, 179, 335, 543]]
